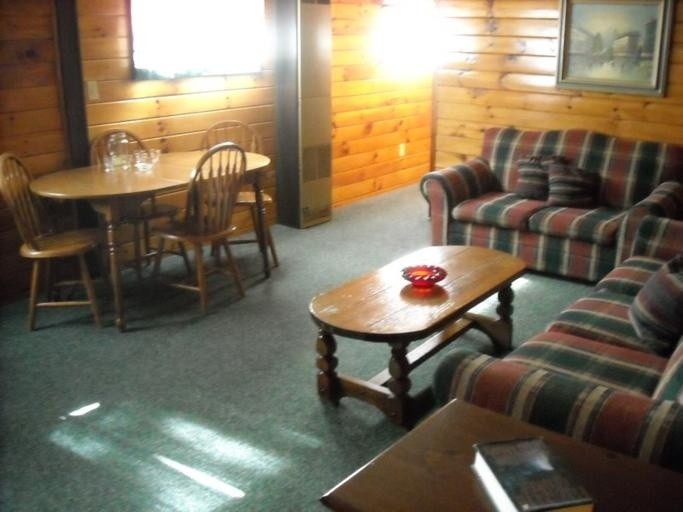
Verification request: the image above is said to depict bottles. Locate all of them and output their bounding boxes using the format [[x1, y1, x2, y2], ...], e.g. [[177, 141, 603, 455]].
[[102, 131, 130, 172]]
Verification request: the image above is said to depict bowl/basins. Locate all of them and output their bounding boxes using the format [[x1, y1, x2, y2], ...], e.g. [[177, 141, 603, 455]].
[[403, 264, 445, 287], [133, 149, 161, 167]]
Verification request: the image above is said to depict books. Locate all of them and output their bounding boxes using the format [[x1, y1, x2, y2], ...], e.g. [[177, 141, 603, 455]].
[[468, 433, 596, 511]]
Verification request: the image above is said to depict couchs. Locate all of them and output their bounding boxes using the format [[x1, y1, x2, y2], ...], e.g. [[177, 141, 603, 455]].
[[418, 127, 683, 285], [432, 214, 682, 467]]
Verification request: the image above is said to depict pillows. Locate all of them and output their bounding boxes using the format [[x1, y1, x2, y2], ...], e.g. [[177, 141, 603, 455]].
[[517, 156, 598, 207], [628, 254, 682, 355]]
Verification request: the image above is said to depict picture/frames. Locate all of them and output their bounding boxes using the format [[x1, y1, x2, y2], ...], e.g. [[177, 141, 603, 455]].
[[555, 0, 677, 99]]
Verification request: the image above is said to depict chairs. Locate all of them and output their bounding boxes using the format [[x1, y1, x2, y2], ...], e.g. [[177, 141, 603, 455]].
[[0, 120, 281, 332]]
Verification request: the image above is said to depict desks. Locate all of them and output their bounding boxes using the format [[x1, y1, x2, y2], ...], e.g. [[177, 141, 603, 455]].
[[320, 397, 640, 512]]
